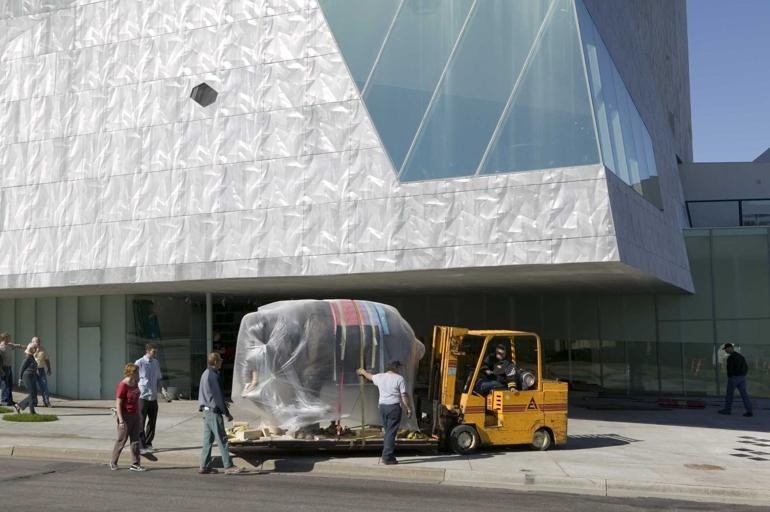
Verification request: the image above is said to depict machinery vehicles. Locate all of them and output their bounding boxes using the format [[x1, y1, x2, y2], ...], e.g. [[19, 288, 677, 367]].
[[228, 324, 569, 453]]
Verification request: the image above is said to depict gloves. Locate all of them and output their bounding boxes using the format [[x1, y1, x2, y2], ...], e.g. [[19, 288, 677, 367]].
[[405, 407, 412, 419], [46, 368, 51, 376], [358, 367, 366, 378]]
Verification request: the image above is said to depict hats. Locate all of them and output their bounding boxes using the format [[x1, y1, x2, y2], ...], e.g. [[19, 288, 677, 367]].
[[722, 342, 732, 350], [387, 359, 403, 369]]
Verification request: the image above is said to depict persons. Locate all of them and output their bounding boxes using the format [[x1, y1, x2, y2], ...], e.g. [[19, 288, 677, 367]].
[[354, 359, 414, 466], [716, 342, 755, 418], [108, 362, 150, 475], [194, 352, 246, 475], [0, 331, 55, 416], [473, 343, 521, 397], [134, 341, 173, 457]]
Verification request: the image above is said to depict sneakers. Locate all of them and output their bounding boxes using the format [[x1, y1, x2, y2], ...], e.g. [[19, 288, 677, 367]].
[[109, 459, 119, 471], [224, 465, 245, 474], [128, 463, 148, 472], [198, 466, 218, 475], [139, 448, 153, 455]]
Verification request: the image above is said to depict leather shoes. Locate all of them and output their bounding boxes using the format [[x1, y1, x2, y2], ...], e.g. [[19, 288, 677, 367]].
[[718, 409, 731, 415], [383, 457, 398, 465], [12, 403, 20, 414], [743, 411, 753, 417]]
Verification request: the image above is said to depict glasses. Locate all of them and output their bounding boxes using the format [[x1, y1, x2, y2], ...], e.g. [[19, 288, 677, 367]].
[[216, 358, 223, 362]]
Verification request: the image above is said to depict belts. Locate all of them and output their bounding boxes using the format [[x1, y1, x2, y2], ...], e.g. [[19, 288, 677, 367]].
[[202, 407, 219, 414]]
